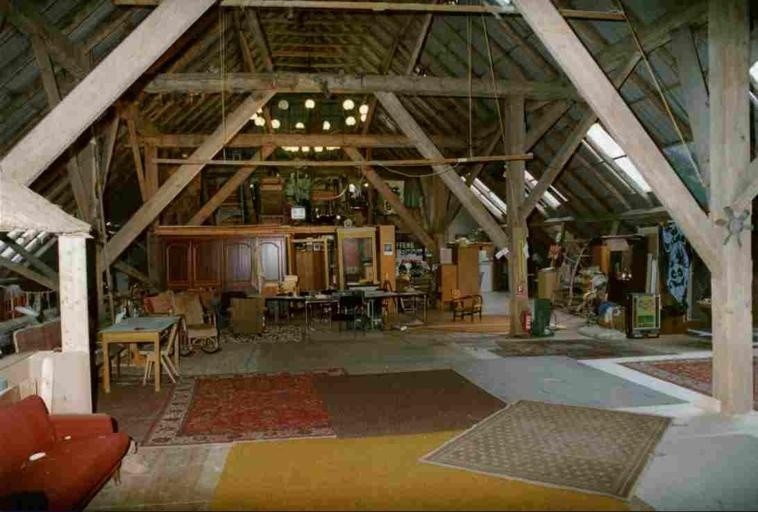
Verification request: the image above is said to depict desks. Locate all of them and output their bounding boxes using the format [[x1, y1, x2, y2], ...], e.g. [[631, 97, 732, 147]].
[[248, 293, 429, 328], [102, 314, 180, 393]]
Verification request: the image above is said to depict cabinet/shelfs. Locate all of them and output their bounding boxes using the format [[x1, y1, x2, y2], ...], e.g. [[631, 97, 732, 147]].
[[159, 233, 287, 325], [335, 226, 377, 292]]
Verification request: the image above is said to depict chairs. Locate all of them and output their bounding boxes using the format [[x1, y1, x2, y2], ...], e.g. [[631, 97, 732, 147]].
[[329, 295, 364, 334], [450, 294, 482, 322], [171, 291, 222, 356], [139, 319, 179, 386]]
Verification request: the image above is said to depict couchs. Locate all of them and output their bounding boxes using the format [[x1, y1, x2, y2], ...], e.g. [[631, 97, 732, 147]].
[[1, 393, 131, 512]]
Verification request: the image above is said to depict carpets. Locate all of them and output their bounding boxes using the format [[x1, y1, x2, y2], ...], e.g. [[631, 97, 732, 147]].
[[617, 354, 757, 412], [94, 355, 673, 509]]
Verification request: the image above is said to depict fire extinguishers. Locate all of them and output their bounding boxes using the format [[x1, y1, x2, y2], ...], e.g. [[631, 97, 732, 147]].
[[518, 308, 532, 332]]
